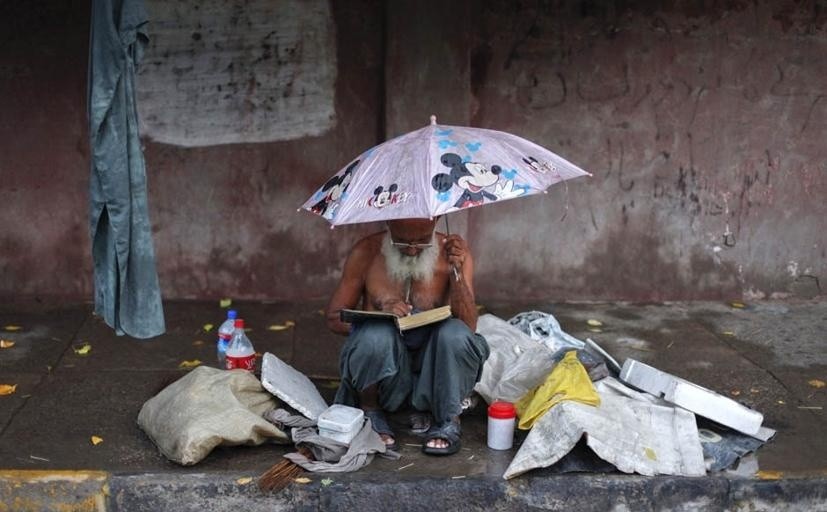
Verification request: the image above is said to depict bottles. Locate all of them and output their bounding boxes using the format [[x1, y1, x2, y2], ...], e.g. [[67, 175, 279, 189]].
[[217, 309, 238, 369], [487, 400, 517, 450], [224, 319, 256, 375]]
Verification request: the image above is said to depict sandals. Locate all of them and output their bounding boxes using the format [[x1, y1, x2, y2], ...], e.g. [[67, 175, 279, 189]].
[[364, 408, 400, 451], [422, 416, 463, 455]]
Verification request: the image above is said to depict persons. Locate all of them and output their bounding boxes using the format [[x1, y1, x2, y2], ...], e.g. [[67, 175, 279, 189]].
[[323, 214, 490, 455]]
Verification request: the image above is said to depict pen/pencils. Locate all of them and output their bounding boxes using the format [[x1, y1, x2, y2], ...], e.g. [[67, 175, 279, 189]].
[[404, 285, 411, 304]]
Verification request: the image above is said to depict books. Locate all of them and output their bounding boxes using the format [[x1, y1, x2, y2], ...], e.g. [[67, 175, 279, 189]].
[[340, 304, 452, 336]]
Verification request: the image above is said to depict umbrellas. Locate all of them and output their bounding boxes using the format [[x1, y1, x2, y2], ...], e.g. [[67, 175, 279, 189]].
[[296, 113, 593, 284]]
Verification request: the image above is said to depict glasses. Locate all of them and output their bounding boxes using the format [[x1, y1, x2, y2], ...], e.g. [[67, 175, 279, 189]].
[[387, 225, 436, 249]]
